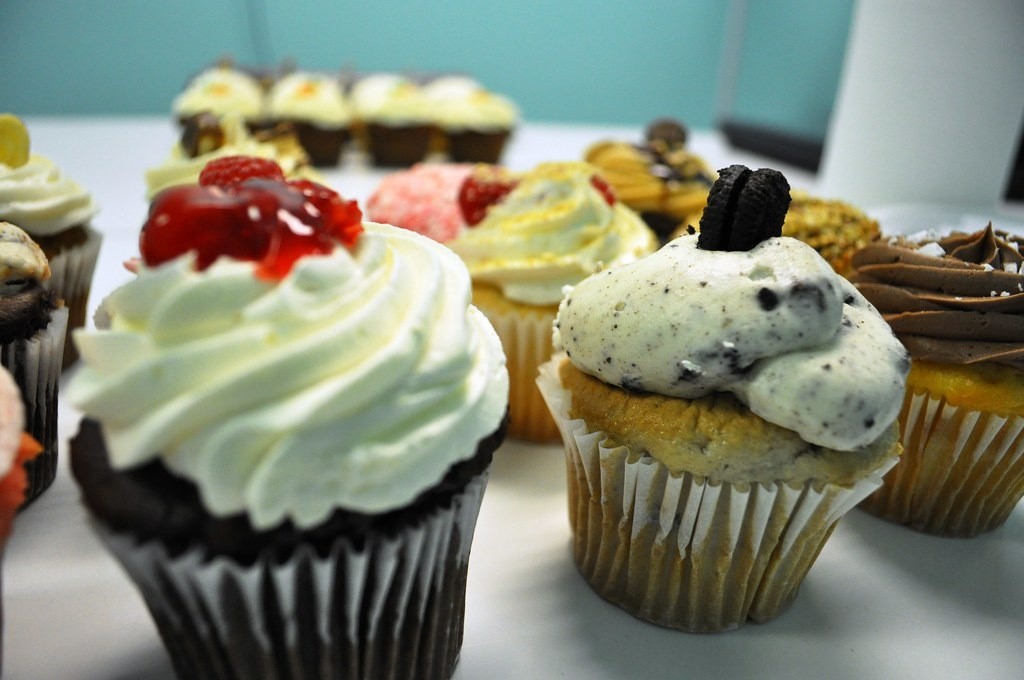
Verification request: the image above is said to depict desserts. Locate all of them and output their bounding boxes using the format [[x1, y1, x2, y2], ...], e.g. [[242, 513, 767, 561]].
[[0, 65, 1024, 680]]
